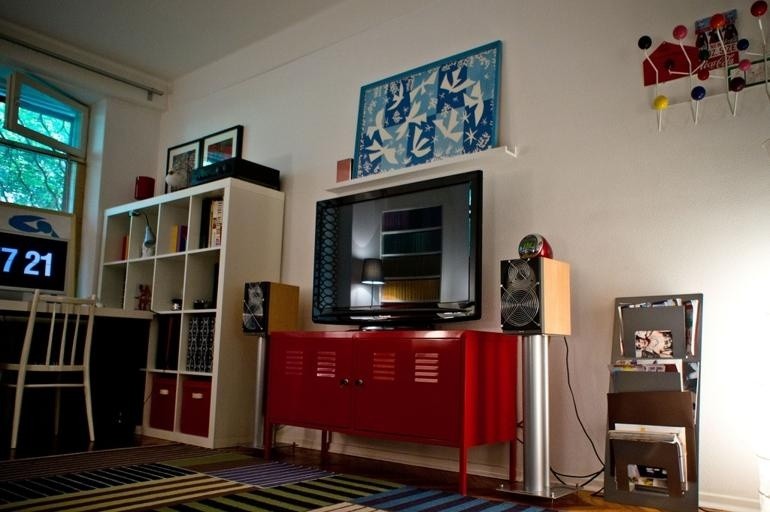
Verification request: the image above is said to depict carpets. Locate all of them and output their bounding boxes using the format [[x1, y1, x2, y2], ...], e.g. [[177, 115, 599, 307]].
[[0, 453, 558, 512]]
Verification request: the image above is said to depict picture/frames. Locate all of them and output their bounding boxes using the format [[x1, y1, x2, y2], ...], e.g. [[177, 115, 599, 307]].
[[200, 125, 242, 166], [166, 140, 198, 192]]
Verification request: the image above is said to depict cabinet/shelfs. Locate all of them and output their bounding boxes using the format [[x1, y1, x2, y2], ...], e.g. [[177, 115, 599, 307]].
[[98, 178, 285, 448], [263, 323, 519, 497]]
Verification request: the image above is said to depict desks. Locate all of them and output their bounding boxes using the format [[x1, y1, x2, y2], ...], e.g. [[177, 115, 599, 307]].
[[0, 288, 150, 452]]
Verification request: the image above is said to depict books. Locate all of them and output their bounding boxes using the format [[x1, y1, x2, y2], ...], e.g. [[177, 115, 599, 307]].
[[171, 224, 188, 253], [617, 299, 699, 357], [607, 423, 689, 495], [607, 358, 700, 425], [199, 196, 223, 248]]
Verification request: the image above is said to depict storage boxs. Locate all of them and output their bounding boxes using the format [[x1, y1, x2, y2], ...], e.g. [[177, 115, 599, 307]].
[[155, 314, 182, 371], [150, 376, 176, 433], [188, 315, 215, 373], [182, 379, 209, 438]]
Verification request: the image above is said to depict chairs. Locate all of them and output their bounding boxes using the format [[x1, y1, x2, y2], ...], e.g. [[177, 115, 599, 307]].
[[6, 287, 97, 447]]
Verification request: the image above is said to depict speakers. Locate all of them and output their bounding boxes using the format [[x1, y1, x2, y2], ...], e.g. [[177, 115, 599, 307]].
[[501, 257, 572, 334], [243, 282, 298, 334]]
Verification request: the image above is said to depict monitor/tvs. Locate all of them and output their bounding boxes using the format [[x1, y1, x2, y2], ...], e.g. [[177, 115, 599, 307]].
[[0, 228, 70, 292], [312, 170, 482, 321]]
